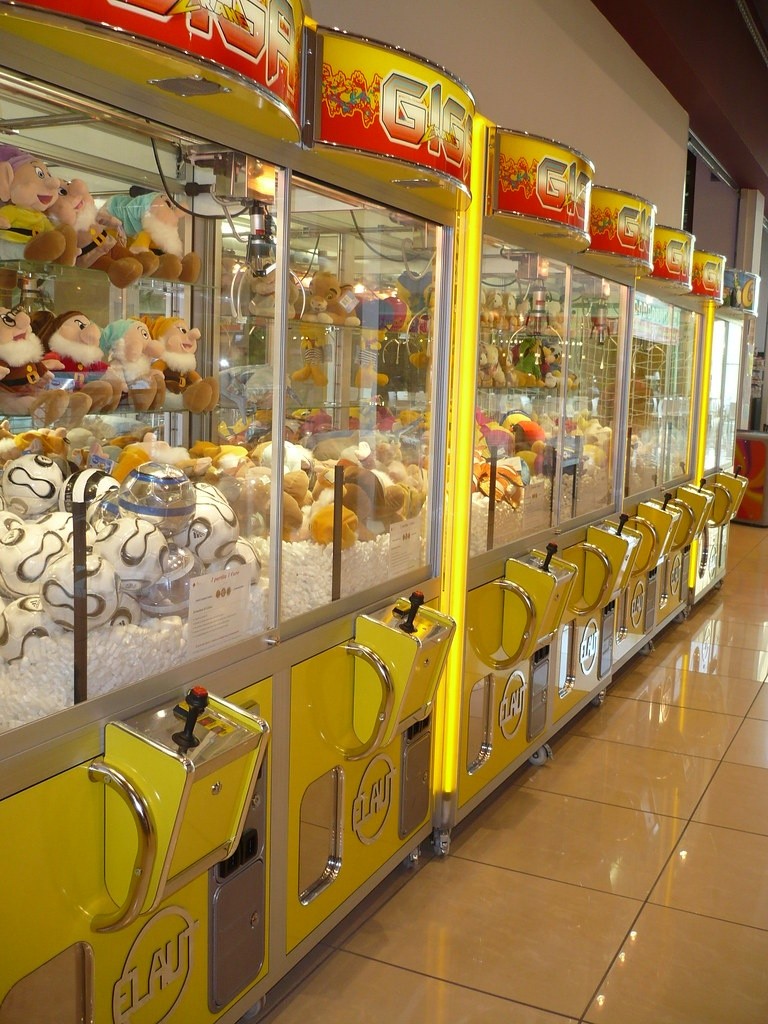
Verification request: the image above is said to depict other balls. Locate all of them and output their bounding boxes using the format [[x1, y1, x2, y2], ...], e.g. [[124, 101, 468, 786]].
[[0, 451, 262, 668]]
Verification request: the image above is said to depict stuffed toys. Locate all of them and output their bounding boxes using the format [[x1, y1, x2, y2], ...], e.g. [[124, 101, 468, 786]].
[[0, 394, 694, 555], [0, 140, 201, 290], [0, 306, 220, 429], [248, 240, 583, 392]]
